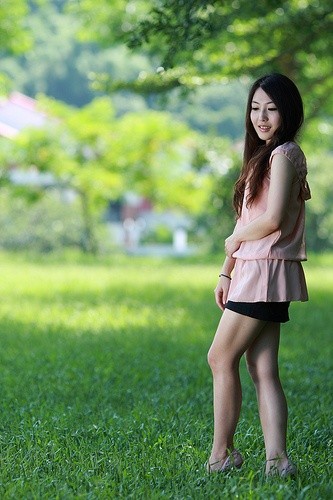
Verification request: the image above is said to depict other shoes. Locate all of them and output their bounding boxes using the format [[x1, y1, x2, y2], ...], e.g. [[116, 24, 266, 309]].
[[265, 460, 297, 482], [196, 448, 243, 474]]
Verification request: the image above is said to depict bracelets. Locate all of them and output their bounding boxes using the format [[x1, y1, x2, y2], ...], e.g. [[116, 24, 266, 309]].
[[218, 273, 232, 280]]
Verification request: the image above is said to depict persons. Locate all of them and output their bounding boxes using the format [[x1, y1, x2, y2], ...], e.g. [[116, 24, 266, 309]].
[[206, 73, 312, 481]]
